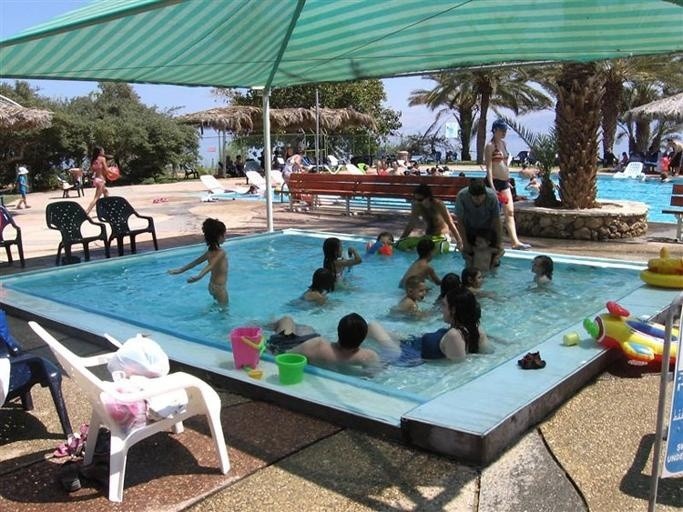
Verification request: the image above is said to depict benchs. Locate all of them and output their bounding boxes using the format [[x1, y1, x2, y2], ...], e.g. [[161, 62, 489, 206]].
[[661, 181, 682, 243], [281, 171, 485, 217]]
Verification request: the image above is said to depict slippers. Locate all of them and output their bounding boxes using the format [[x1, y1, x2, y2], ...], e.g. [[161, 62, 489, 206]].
[[512, 243, 531, 250]]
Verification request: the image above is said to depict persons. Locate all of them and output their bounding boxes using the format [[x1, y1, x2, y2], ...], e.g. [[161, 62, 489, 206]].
[[531, 256, 553, 284], [484, 121, 531, 250], [270, 231, 505, 365], [604, 138, 683, 181], [219, 144, 464, 202], [170, 217, 229, 304], [520, 161, 542, 195], [454, 181, 503, 269], [399, 184, 463, 248], [15, 167, 31, 209], [57, 146, 120, 215]]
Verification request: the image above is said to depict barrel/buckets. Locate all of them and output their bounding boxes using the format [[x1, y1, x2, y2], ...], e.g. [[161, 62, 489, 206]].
[[229, 326, 262, 370], [277, 352, 307, 387]]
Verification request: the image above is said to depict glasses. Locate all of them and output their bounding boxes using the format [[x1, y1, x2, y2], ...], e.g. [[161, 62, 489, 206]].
[[414, 197, 425, 201]]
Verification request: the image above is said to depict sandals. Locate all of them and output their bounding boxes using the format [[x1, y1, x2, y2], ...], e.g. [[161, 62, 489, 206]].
[[58, 461, 81, 491], [54, 424, 90, 460]]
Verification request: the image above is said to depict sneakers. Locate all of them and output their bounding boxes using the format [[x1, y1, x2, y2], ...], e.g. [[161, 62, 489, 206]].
[[518, 352, 545, 369]]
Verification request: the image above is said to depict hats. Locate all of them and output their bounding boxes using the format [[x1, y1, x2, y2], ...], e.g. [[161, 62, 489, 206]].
[[493, 119, 507, 129]]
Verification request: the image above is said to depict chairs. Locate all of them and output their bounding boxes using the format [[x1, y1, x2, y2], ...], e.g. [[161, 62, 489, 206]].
[[0, 309, 74, 443], [611, 160, 645, 182], [345, 162, 363, 174], [44, 201, 109, 266], [0, 205, 26, 268], [25, 319, 231, 504], [198, 166, 289, 200], [94, 196, 157, 256]]
